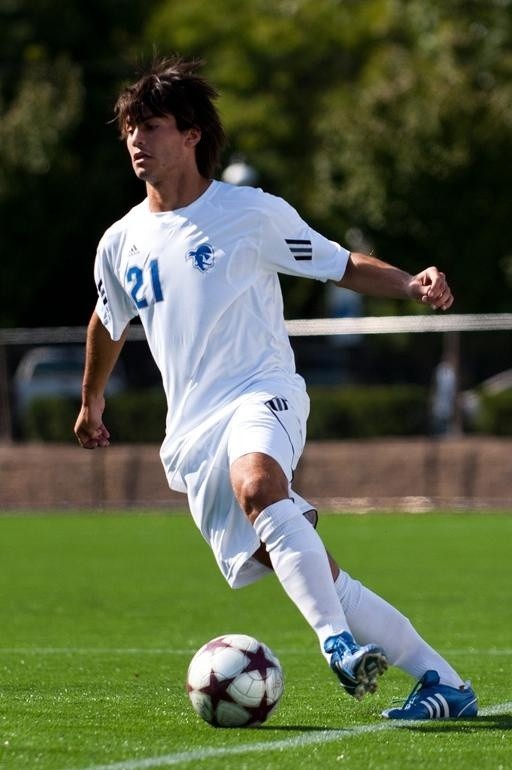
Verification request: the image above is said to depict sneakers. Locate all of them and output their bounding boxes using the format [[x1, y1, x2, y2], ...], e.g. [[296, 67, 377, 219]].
[[323, 630, 389, 702], [382, 670, 479, 721]]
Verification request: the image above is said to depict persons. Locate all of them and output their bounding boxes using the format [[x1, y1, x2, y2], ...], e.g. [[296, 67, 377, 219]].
[[71, 52, 481, 720]]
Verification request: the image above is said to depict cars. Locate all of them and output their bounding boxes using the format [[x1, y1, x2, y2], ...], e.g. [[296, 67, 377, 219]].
[[14, 344, 125, 400]]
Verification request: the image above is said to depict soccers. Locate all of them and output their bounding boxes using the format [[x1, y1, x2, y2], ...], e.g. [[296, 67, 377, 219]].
[[187, 634, 282, 727]]
